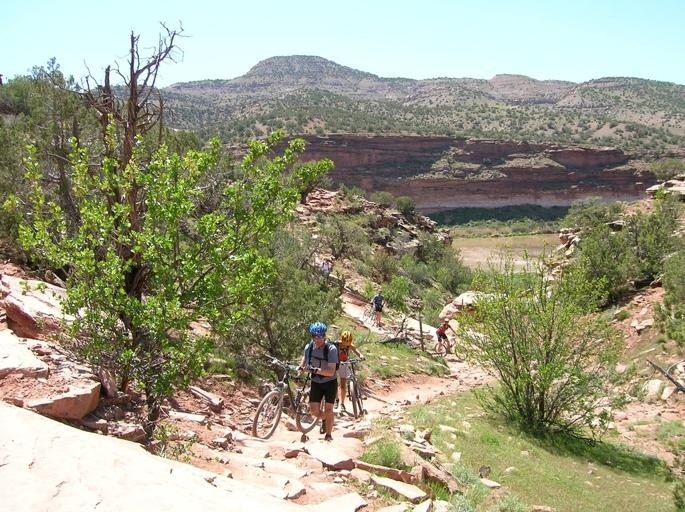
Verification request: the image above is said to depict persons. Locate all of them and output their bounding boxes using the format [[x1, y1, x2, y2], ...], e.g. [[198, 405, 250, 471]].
[[299, 321, 339, 441], [333, 331, 365, 412], [373, 291, 385, 328], [436, 318, 459, 352]]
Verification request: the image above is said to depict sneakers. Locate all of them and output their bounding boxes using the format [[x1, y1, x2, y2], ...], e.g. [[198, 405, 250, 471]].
[[320, 420, 333, 441]]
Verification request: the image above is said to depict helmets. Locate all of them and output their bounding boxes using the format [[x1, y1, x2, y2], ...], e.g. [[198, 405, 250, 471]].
[[309, 322, 327, 336], [341, 331, 352, 345]]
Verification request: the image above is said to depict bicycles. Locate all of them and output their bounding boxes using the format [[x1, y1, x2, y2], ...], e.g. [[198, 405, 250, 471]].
[[434, 336, 468, 361], [363, 300, 385, 327], [252, 352, 324, 440], [335, 357, 367, 419]]
